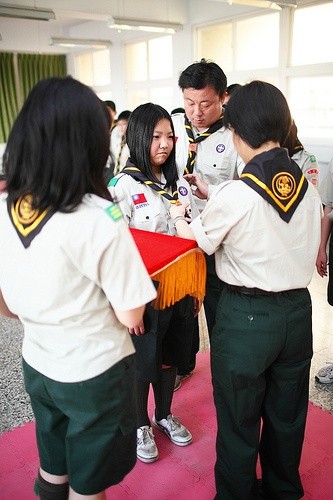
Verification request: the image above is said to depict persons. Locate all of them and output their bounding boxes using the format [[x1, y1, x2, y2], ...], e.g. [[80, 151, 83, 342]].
[[170, 57, 247, 394], [1, 76, 156, 499], [104, 96, 192, 185], [168, 80, 333, 500], [110, 103, 205, 464]]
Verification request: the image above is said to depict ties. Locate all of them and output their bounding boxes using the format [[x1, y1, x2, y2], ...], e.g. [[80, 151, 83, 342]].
[[240, 147, 309, 223], [116, 134, 127, 175], [183, 113, 226, 176], [120, 158, 191, 224], [6, 189, 60, 249]]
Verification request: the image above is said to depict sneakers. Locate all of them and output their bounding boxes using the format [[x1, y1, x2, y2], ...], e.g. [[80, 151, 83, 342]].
[[137, 425, 159, 464], [315, 362, 333, 384], [151, 408, 193, 447]]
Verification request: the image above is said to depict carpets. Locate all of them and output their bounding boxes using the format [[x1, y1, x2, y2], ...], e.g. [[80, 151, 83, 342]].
[[0, 351, 333, 500]]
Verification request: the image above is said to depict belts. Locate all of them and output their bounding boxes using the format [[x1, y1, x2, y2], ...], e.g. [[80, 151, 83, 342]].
[[225, 282, 308, 296]]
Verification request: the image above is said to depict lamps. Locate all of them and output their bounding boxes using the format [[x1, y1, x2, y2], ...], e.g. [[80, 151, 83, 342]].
[[0, 0, 55, 21], [108, 0, 184, 34], [49, 36, 112, 50], [218, 0, 298, 12]]
